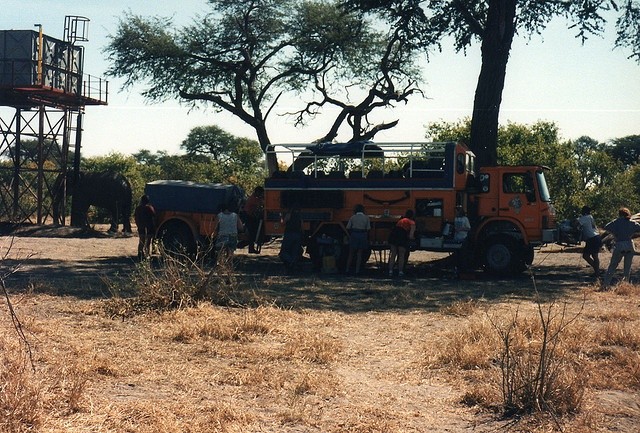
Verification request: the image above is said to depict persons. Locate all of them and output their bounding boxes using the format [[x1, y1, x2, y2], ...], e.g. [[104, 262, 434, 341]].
[[243, 186, 264, 253], [279, 197, 306, 264], [345, 204, 371, 278], [135, 195, 157, 259], [450, 205, 471, 269], [573, 206, 603, 278], [388, 209, 417, 277], [595, 207, 640, 286], [215, 203, 243, 285]]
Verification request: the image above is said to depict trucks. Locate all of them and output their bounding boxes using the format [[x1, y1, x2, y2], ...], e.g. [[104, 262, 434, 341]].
[[136, 142, 557, 279]]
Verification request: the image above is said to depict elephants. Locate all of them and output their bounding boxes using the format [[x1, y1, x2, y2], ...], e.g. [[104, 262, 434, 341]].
[[52, 169, 132, 233]]
